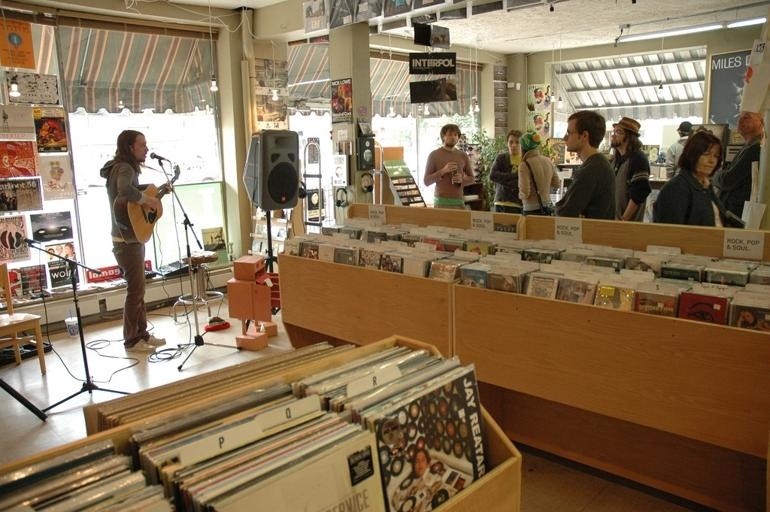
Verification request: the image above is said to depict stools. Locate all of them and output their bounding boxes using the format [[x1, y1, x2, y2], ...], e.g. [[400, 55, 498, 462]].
[[173, 251, 225, 322]]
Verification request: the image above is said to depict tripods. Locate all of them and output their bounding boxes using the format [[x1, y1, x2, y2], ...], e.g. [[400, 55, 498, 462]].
[[42, 265, 134, 413], [246, 212, 280, 335], [176, 221, 243, 370]]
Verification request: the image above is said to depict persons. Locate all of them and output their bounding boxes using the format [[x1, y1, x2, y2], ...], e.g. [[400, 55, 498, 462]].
[[553, 109, 616, 219], [489, 128, 523, 215], [665, 121, 695, 179], [713, 110, 767, 229], [47, 244, 76, 262], [423, 123, 475, 210], [517, 129, 562, 217], [606, 115, 653, 224], [97, 129, 174, 354], [652, 132, 726, 228]]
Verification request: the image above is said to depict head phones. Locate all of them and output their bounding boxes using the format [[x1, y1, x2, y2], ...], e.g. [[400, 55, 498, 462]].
[[334, 187, 349, 208], [360, 172, 374, 193]]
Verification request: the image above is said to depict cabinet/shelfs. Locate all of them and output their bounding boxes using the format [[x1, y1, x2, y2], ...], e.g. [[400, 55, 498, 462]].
[[226, 255, 280, 335]]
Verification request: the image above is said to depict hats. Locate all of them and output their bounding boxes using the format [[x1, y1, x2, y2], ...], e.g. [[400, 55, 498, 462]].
[[613, 116, 641, 137], [677, 121, 695, 134], [205, 317, 230, 332], [519, 129, 541, 151]]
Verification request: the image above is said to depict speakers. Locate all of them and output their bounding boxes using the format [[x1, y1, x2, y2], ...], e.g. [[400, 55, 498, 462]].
[[248, 130, 299, 211]]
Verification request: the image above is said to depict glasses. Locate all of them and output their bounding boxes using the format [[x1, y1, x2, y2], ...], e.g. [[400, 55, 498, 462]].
[[566, 129, 583, 136], [611, 129, 627, 135]]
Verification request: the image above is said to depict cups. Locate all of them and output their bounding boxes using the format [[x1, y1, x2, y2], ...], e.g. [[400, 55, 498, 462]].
[[65, 317, 79, 338]]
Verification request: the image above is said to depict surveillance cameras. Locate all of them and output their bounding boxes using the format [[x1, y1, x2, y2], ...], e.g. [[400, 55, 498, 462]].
[[620, 24, 630, 32]]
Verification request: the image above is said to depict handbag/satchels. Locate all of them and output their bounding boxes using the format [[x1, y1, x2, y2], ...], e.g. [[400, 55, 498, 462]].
[[541, 206, 552, 215]]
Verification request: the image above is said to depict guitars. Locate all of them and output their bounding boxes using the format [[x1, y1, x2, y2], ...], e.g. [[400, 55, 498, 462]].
[[113, 165, 180, 244]]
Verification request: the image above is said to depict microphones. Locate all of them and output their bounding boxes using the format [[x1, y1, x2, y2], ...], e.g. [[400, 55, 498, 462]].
[[23, 237, 36, 243], [150, 153, 166, 161]]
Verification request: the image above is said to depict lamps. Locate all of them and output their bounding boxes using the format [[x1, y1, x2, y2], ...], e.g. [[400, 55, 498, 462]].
[[550, 49, 557, 104], [656, 38, 666, 97], [271, 41, 280, 102], [468, 41, 480, 118], [209, 6, 219, 93], [557, 49, 564, 110], [0, 9, 22, 98]]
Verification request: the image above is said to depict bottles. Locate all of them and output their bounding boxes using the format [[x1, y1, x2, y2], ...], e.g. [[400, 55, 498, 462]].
[[452, 169, 460, 187]]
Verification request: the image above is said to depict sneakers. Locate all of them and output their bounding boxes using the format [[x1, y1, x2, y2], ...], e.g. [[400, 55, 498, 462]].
[[125, 338, 156, 353], [146, 335, 166, 347]]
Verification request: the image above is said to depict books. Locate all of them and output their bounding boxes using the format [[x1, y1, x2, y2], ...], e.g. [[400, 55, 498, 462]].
[[392, 177, 423, 207], [283, 217, 770, 335], [1, 339, 488, 512]]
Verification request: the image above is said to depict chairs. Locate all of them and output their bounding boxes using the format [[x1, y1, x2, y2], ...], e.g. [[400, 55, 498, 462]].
[[0, 260, 47, 375]]
